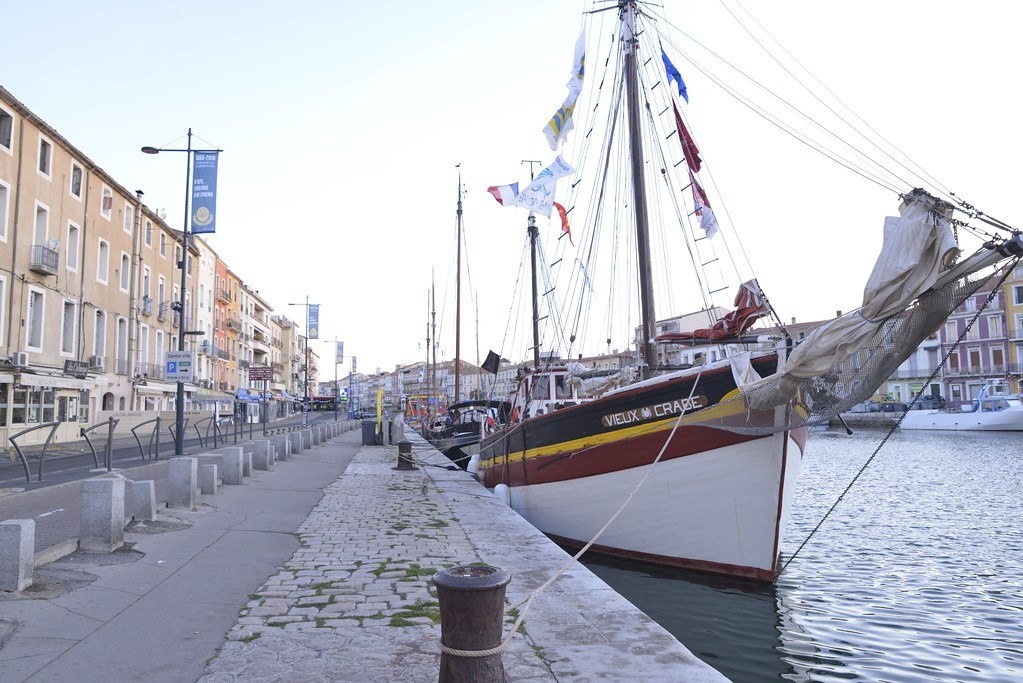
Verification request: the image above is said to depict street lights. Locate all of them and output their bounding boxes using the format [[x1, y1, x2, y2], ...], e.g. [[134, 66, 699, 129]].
[[289, 302, 309, 397], [324, 340, 339, 422], [141, 146, 191, 454]]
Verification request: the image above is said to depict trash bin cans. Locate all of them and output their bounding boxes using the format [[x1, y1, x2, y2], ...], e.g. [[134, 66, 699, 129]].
[[361, 420, 377, 445]]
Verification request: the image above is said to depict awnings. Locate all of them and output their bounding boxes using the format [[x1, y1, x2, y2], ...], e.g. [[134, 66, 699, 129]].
[[15, 372, 94, 389]]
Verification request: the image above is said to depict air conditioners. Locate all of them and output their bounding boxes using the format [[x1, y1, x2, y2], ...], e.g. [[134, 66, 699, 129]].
[[12, 352, 30, 367], [91, 355, 104, 367]]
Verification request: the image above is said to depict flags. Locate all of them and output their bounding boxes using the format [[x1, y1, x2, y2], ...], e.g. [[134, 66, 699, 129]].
[[487, 183, 518, 206], [419, 365, 425, 383], [511, 153, 576, 220], [673, 99, 702, 173], [542, 23, 586, 152], [688, 170, 718, 239], [553, 202, 574, 247], [661, 45, 688, 105]]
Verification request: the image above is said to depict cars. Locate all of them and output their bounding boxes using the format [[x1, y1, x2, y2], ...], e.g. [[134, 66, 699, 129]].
[[913, 395, 946, 409]]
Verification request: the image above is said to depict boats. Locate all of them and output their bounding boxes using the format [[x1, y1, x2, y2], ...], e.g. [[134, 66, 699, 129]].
[[892, 378, 1023, 431]]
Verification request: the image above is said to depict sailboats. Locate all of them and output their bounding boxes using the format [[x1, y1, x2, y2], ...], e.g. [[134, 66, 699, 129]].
[[468, 161, 619, 472], [478, 0, 1023, 582], [421, 163, 511, 471]]
